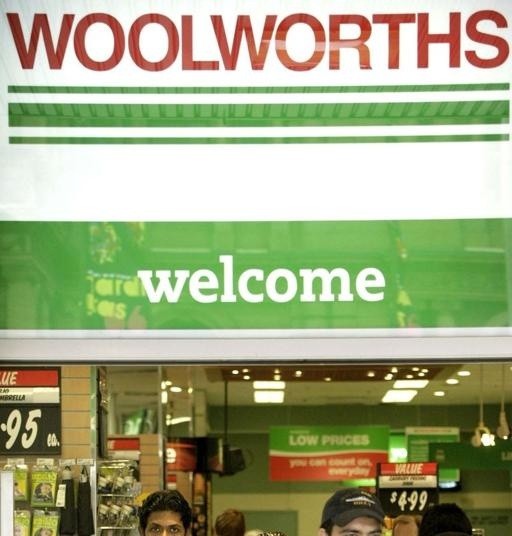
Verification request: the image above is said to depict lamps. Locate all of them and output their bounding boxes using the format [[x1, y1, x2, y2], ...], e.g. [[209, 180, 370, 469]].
[[470, 363, 511, 448]]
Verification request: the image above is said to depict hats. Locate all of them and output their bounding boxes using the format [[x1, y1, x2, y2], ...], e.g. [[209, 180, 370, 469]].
[[322, 487, 388, 529]]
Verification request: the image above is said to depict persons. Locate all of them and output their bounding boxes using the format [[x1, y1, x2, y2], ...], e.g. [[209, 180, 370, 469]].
[[13, 482, 53, 536], [136, 489, 192, 536], [213, 507, 246, 536], [318, 487, 388, 536]]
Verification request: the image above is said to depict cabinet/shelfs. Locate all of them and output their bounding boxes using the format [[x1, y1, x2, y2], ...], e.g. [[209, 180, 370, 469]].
[[95, 460, 140, 536]]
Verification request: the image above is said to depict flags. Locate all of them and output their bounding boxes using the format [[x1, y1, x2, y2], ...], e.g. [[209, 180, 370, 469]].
[[268, 423, 391, 484]]
